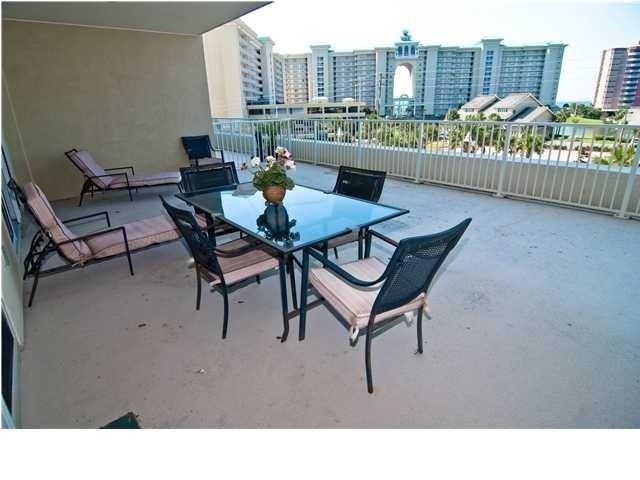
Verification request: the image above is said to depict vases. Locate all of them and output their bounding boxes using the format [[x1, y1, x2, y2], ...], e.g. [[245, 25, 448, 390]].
[[260, 184, 287, 204]]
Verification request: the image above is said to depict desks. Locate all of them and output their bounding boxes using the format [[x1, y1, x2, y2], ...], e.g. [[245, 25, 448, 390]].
[[173, 180, 409, 342]]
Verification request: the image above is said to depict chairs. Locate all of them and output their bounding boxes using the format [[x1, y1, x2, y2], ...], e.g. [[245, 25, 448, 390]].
[[298, 217, 473, 394], [158, 192, 298, 341], [311, 164, 388, 267], [7, 148, 219, 308], [179, 161, 243, 245], [180, 135, 225, 168]]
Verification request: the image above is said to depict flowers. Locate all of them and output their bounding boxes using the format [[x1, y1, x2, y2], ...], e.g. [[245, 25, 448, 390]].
[[241, 146, 297, 179]]
